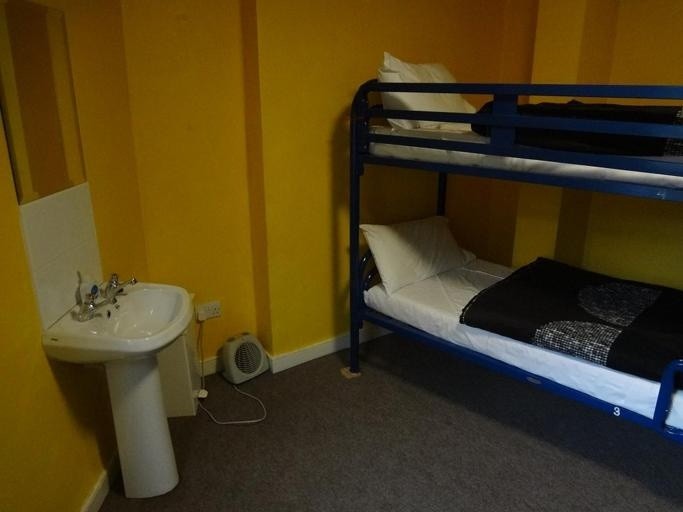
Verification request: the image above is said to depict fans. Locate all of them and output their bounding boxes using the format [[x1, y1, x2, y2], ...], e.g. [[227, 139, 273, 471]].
[[220, 331, 271, 385]]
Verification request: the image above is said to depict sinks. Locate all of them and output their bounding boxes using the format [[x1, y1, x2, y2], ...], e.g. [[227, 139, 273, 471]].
[[98, 282, 193, 352]]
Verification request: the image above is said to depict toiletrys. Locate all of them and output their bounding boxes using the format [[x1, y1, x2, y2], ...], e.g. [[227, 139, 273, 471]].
[[77, 268, 102, 304]]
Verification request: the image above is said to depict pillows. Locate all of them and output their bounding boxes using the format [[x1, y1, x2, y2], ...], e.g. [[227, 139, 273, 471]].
[[374, 51, 477, 132], [359, 214, 477, 298]]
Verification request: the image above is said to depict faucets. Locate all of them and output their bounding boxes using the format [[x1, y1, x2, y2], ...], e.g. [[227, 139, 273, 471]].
[[77, 292, 108, 322], [104, 271, 137, 303]]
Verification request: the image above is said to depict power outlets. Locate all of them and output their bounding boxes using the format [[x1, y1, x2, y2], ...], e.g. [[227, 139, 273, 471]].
[[192, 299, 221, 323]]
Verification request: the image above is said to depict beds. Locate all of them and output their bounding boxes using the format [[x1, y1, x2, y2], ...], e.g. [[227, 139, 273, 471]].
[[347, 76, 681, 442]]
